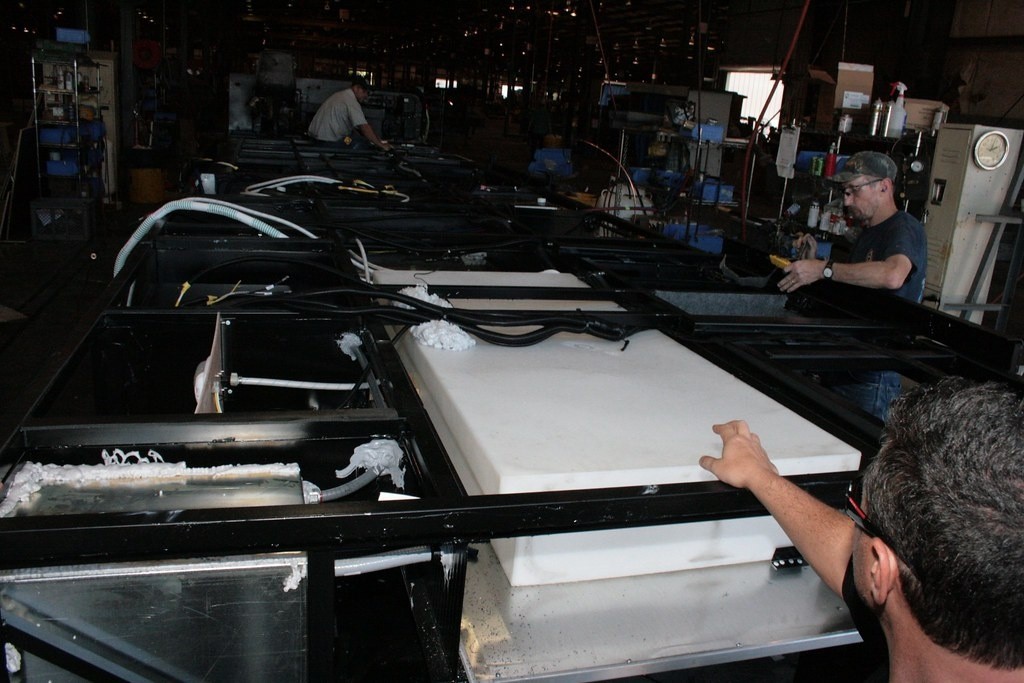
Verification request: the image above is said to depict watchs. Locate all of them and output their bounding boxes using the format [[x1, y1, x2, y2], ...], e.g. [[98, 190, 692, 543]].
[[822, 260, 835, 280]]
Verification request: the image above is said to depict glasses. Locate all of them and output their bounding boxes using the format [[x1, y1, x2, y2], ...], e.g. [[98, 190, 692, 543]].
[[838, 178, 883, 196], [845, 474, 926, 585]]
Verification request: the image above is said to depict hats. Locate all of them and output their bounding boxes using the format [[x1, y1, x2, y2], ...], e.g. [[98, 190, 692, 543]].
[[824, 151, 897, 187], [351, 76, 377, 92]]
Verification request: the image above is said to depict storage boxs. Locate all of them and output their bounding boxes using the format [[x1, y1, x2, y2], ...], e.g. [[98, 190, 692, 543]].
[[806, 61, 875, 112], [628, 167, 670, 184], [667, 174, 735, 201], [904, 98, 950, 132], [815, 112, 869, 135], [680, 122, 725, 142], [663, 224, 724, 255]]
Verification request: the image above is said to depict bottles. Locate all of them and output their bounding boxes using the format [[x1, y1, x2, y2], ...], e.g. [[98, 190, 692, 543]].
[[823, 142, 838, 177], [807, 202, 819, 228], [868, 98, 892, 136]]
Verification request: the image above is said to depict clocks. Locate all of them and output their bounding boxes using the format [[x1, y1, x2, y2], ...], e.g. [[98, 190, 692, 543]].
[[974, 130, 1010, 170]]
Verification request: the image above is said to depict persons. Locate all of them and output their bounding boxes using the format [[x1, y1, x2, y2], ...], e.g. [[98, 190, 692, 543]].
[[307, 81, 395, 151], [777, 151, 928, 304], [700, 376, 1024, 683], [527, 104, 552, 143]]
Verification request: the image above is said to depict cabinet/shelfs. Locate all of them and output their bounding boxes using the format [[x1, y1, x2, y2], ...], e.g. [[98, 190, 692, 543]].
[[615, 127, 752, 244], [31, 56, 109, 240], [919, 124, 1024, 327]]
